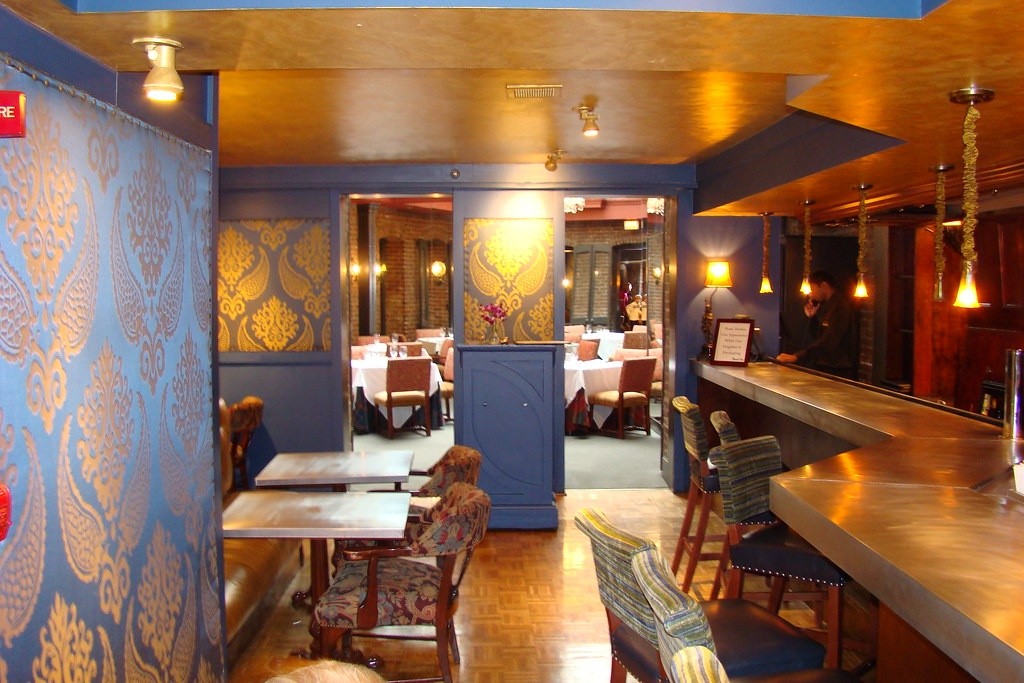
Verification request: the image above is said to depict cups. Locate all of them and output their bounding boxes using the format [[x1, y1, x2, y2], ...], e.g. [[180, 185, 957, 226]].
[[440, 327, 446, 337], [373, 333, 380, 344], [448, 328, 454, 339], [585, 324, 593, 334], [399, 346, 407, 358], [596, 325, 602, 333], [390, 345, 398, 360], [391, 333, 398, 344]]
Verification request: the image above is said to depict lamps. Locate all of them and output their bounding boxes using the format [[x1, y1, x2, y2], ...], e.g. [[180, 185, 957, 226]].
[[758, 211, 773, 294], [545, 149, 564, 171], [927, 162, 957, 302], [430, 256, 447, 283], [949, 87, 994, 308], [132, 37, 185, 102], [572, 106, 599, 136], [703, 261, 734, 361], [800, 200, 815, 295], [854, 184, 874, 298]]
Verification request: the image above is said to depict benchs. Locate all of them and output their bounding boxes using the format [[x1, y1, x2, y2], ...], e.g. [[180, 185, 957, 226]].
[[219, 398, 306, 674]]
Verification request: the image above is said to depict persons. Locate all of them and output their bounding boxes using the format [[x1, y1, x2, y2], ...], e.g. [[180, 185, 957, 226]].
[[624, 282, 632, 304], [776, 270, 856, 377]]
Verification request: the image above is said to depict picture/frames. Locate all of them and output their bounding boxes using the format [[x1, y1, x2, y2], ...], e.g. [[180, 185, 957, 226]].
[[710, 318, 754, 367]]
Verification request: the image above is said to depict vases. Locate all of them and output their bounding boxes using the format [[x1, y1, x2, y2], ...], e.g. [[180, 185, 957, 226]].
[[487, 318, 509, 346]]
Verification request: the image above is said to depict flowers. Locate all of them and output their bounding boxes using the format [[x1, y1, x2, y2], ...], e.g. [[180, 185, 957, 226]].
[[478, 303, 509, 325]]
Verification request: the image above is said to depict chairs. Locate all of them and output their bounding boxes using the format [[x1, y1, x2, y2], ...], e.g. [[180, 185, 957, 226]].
[[564, 321, 662, 441], [359, 328, 455, 439], [314, 444, 491, 683], [228, 395, 263, 493], [573, 505, 863, 683]]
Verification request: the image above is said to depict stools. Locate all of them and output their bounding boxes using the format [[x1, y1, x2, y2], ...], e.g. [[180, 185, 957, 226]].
[[670, 396, 880, 671]]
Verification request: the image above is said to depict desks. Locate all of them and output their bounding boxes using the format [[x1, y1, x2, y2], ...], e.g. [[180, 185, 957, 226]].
[[582, 332, 625, 360], [416, 337, 446, 363], [255, 450, 417, 579], [351, 355, 445, 434], [223, 491, 412, 670], [564, 360, 623, 435]]
